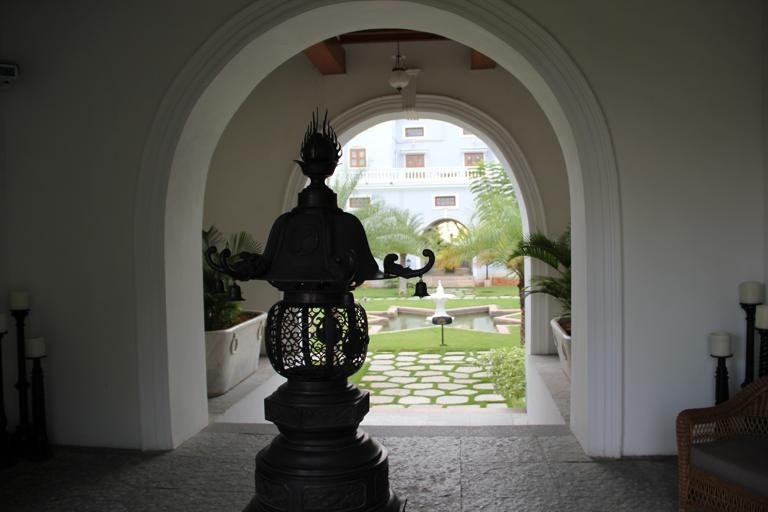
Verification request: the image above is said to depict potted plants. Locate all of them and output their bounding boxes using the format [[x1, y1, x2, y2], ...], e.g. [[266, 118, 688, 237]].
[[201, 226, 269, 397], [510, 225, 571, 377]]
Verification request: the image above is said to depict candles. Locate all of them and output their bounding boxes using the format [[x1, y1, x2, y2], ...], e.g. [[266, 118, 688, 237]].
[[707, 330, 730, 356], [737, 280, 767, 330], [23, 337, 45, 357], [9, 288, 29, 309]]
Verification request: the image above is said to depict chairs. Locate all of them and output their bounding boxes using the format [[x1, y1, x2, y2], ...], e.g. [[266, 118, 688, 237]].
[[674, 375, 767, 512]]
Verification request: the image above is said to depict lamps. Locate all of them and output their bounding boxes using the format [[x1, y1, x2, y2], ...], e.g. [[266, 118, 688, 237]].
[[388, 36, 410, 95]]
